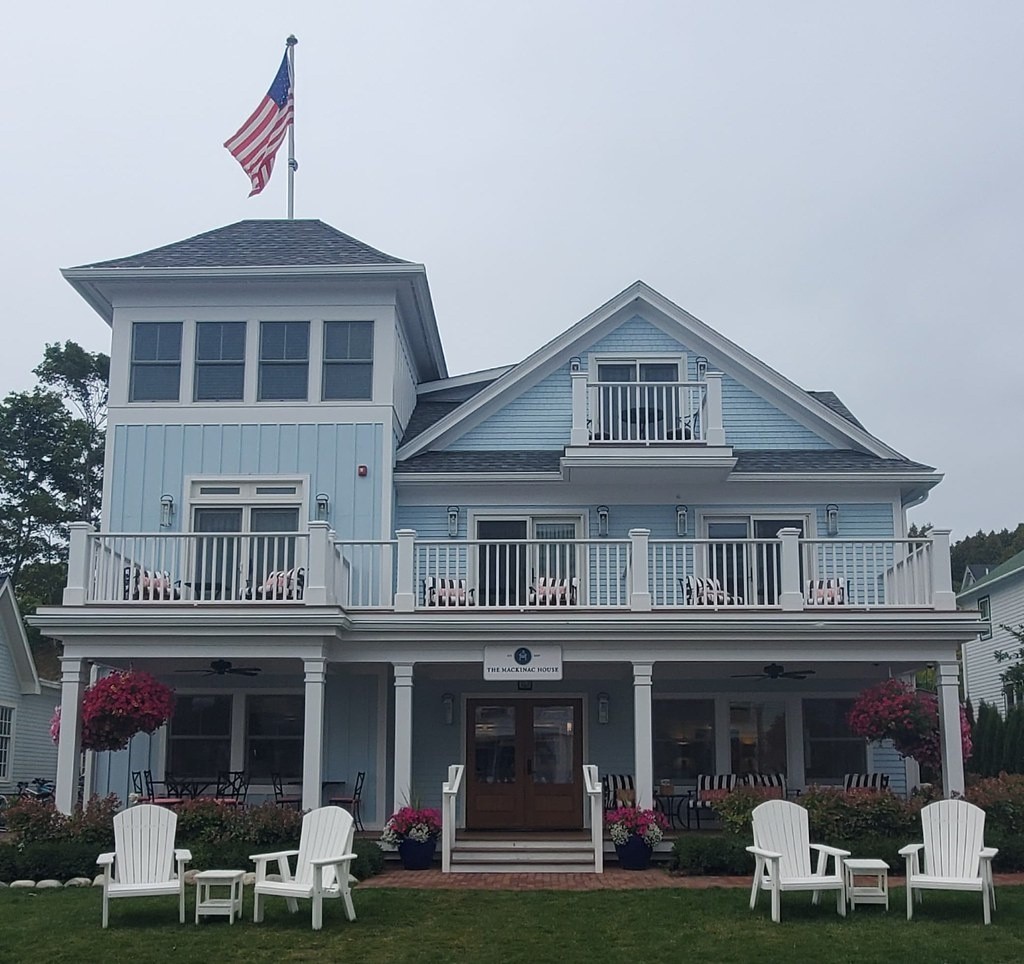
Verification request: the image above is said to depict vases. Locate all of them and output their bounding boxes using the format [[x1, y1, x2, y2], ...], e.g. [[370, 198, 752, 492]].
[[617, 831, 653, 869], [399, 833, 440, 871]]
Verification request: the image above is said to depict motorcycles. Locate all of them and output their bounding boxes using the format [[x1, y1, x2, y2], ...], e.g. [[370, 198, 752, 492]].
[[32, 777, 56, 798], [16, 782, 55, 807]]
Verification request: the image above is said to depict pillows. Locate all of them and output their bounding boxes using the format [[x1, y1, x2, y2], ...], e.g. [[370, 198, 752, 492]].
[[698, 786, 729, 800], [141, 575, 167, 585], [267, 575, 284, 586], [539, 584, 564, 594], [437, 587, 463, 595], [616, 789, 635, 801], [699, 587, 727, 598], [812, 587, 838, 598]]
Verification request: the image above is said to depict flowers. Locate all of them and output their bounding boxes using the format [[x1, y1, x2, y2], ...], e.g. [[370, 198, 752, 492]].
[[381, 806, 440, 848], [605, 806, 667, 847], [48, 673, 174, 754]]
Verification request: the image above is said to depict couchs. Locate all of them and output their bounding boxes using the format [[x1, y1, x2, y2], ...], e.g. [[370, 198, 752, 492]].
[[676, 577, 742, 605], [804, 577, 851, 606], [528, 577, 580, 605], [123, 568, 180, 601], [423, 577, 473, 605], [237, 567, 307, 602]]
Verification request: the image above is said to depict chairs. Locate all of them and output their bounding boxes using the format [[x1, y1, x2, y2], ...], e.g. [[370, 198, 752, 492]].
[[329, 772, 366, 832], [744, 773, 800, 805], [585, 415, 612, 440], [97, 804, 193, 928], [898, 798, 1000, 924], [842, 771, 889, 799], [602, 774, 636, 809], [666, 409, 701, 439], [249, 805, 359, 931], [745, 799, 851, 922], [271, 772, 302, 812], [678, 774, 740, 829], [132, 767, 251, 809]]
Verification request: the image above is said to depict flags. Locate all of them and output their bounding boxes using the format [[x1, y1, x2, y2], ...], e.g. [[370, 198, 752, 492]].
[[222, 46, 294, 198]]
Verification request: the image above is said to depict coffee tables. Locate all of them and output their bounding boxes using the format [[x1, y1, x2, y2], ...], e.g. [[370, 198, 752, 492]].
[[185, 582, 229, 599]]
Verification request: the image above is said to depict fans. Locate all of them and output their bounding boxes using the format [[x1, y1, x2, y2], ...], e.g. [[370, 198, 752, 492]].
[[730, 663, 816, 680], [174, 659, 261, 678]]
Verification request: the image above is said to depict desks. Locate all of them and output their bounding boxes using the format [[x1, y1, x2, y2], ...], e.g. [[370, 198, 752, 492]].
[[652, 793, 688, 830], [172, 778, 227, 800], [286, 780, 345, 811], [193, 870, 247, 924], [844, 859, 889, 910], [620, 407, 662, 439]]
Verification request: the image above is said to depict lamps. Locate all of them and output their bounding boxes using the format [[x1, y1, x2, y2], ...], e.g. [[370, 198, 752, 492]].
[[827, 502, 838, 536], [599, 694, 609, 725], [315, 491, 330, 520], [597, 506, 611, 535], [696, 357, 708, 381], [569, 357, 581, 373], [676, 504, 689, 537], [159, 493, 174, 526], [443, 693, 454, 724], [446, 506, 460, 536]]
